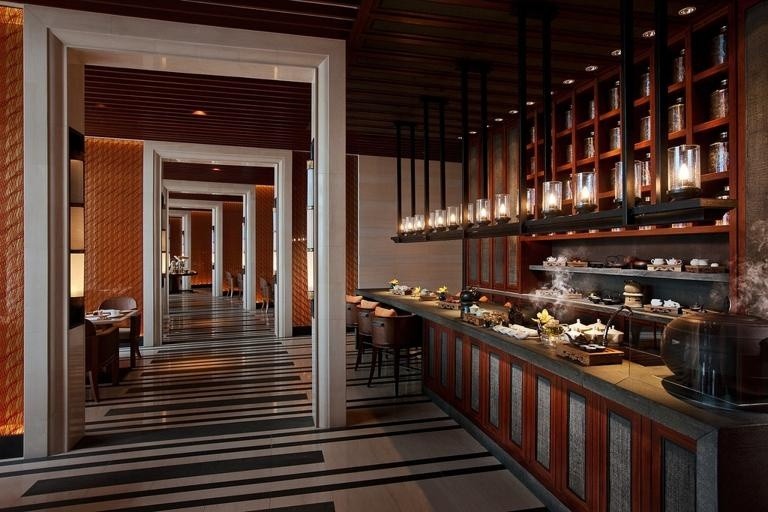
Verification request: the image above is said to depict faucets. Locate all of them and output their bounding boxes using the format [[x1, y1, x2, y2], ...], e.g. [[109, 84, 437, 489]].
[[602, 305, 634, 346]]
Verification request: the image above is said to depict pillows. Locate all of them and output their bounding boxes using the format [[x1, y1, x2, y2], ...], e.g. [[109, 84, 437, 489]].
[[374, 307, 398, 317], [345, 294, 363, 304], [359, 300, 379, 309]]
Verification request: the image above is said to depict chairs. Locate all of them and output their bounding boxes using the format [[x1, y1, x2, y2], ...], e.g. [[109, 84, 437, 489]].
[[345, 293, 364, 333], [96, 294, 144, 361], [83, 317, 118, 407], [355, 299, 405, 378], [258, 275, 274, 313], [223, 269, 238, 298], [236, 270, 243, 299], [366, 304, 426, 400]]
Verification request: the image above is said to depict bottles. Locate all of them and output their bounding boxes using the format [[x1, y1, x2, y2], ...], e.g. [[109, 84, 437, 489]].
[[527, 143, 701, 219], [584, 130, 595, 159], [672, 46, 685, 85], [530, 152, 535, 174], [709, 131, 728, 171], [589, 93, 594, 120], [531, 125, 535, 143], [606, 80, 620, 110], [715, 24, 728, 64], [609, 120, 620, 150], [704, 80, 727, 117], [567, 104, 572, 130], [667, 96, 685, 135], [638, 108, 651, 143], [639, 66, 650, 97]]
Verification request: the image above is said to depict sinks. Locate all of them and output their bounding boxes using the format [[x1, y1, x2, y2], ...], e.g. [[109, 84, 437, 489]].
[[608, 345, 668, 367]]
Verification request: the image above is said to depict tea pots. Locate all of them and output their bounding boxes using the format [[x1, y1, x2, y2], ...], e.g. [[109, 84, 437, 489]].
[[564, 331, 594, 348], [546, 256, 567, 261], [665, 257, 682, 266], [624, 278, 643, 292]]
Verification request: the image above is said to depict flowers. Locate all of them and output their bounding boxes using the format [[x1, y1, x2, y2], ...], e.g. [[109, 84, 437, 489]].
[[387, 278, 448, 301], [531, 308, 561, 330]]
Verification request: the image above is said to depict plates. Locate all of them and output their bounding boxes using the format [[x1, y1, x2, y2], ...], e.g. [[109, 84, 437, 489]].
[[86, 313, 124, 320], [660, 375, 768, 416]]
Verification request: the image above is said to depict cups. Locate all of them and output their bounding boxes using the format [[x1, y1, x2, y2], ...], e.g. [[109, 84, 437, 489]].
[[395, 193, 511, 233], [690, 259, 710, 267], [650, 257, 664, 265], [390, 284, 438, 300], [111, 309, 121, 315], [580, 344, 606, 351]]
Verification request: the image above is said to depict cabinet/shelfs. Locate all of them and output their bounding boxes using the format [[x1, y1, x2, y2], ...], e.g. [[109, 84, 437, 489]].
[[481, 293, 523, 313], [619, 314, 674, 369], [462, 127, 555, 300], [520, 298, 568, 330], [569, 304, 626, 333], [520, 23, 738, 246]]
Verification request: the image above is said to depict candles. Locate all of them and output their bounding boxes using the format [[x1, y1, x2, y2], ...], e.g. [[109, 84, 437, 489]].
[[395, 193, 510, 236], [516, 140, 699, 235]]
